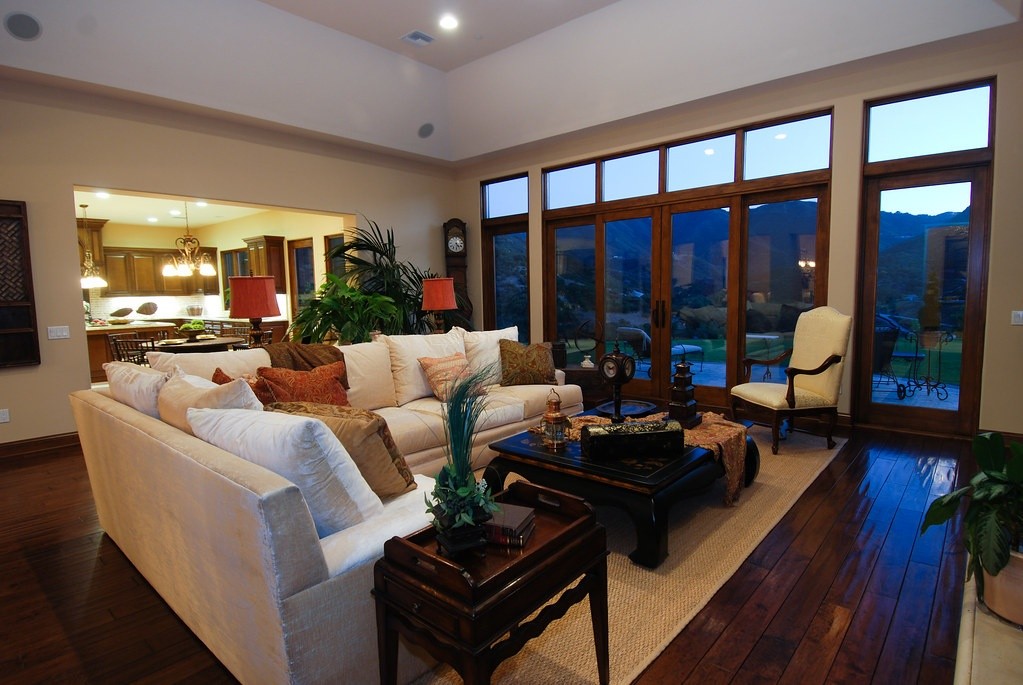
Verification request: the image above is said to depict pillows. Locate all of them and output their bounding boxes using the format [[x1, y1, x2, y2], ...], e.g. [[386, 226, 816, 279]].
[[211, 366, 233, 383], [255, 359, 353, 409], [416, 352, 491, 398], [265, 400, 420, 499], [495, 337, 560, 388], [251, 376, 291, 406]]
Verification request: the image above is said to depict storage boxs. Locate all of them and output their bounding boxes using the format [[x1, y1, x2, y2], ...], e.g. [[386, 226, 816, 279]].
[[578, 417, 685, 459]]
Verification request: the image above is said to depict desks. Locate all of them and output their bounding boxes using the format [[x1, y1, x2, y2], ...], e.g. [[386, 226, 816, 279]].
[[744, 333, 780, 382], [876, 352, 927, 390], [139, 335, 245, 353]]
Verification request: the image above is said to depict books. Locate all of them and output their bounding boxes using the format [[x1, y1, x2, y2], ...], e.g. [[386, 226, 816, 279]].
[[479, 501, 536, 548]]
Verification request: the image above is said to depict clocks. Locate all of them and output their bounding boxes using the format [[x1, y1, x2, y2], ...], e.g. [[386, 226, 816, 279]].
[[442, 217, 469, 257], [596, 337, 638, 424]]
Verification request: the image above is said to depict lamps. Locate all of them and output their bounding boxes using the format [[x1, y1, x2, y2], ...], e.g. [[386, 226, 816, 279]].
[[226, 271, 283, 347], [78, 203, 109, 290], [417, 275, 460, 334], [156, 200, 218, 280]]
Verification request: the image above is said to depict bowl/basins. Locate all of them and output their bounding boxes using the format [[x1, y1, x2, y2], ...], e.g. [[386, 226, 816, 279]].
[[163, 340, 178, 343], [198, 335, 217, 338]]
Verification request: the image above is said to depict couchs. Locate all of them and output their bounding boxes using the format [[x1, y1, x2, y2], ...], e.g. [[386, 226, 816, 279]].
[[84, 318, 588, 473], [71, 369, 445, 685]]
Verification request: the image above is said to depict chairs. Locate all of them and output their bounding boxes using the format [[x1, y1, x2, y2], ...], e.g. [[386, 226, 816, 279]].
[[104, 329, 140, 362], [614, 324, 705, 375], [248, 325, 275, 349], [112, 336, 155, 368], [723, 302, 856, 454]]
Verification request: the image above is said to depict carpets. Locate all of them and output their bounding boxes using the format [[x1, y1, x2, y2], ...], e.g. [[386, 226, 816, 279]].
[[401, 420, 851, 685]]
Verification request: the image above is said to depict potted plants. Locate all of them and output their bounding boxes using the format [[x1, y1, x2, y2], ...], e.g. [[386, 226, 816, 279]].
[[416, 350, 511, 563], [912, 425, 1023, 631], [919, 275, 942, 350], [175, 315, 208, 343]]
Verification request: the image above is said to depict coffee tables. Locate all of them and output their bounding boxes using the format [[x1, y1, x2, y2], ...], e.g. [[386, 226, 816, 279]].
[[482, 398, 766, 570]]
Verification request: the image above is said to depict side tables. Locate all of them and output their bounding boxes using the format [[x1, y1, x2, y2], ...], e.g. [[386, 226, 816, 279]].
[[366, 476, 617, 685]]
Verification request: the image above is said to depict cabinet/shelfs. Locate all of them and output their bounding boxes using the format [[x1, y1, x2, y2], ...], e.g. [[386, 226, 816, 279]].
[[89, 321, 182, 385], [223, 320, 291, 348], [78, 215, 219, 297]]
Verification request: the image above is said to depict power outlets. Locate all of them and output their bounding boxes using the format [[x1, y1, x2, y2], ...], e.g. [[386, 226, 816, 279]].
[[0, 408, 11, 424]]
